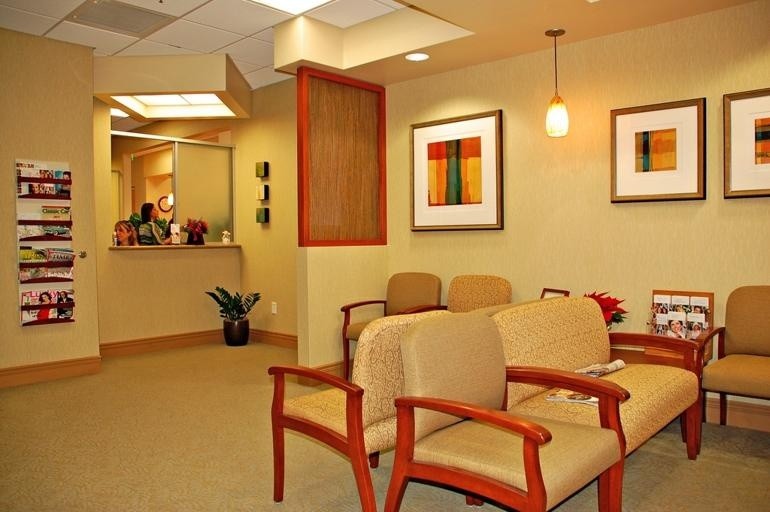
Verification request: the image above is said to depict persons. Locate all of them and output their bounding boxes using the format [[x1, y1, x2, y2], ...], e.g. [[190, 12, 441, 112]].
[[114, 220, 140, 246], [138, 202, 172, 245]]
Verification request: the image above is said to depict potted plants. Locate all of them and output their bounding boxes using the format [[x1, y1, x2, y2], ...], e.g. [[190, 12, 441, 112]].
[[204, 285, 262, 347]]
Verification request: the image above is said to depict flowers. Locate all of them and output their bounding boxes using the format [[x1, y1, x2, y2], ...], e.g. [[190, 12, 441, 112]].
[[183, 217, 209, 241]]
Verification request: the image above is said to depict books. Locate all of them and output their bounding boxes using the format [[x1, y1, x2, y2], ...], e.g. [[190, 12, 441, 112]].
[[651, 291, 708, 350], [16, 160, 75, 324], [546, 385, 600, 407], [573, 358, 625, 378]]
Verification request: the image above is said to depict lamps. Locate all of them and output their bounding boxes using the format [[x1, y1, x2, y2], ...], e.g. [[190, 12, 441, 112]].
[[544, 27, 568, 138]]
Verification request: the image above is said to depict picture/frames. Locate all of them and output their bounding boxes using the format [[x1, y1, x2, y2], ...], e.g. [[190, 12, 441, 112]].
[[609, 86, 770, 205], [409, 107, 503, 231]]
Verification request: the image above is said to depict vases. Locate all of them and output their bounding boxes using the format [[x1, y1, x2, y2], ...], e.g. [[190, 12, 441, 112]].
[[187, 233, 204, 245]]
